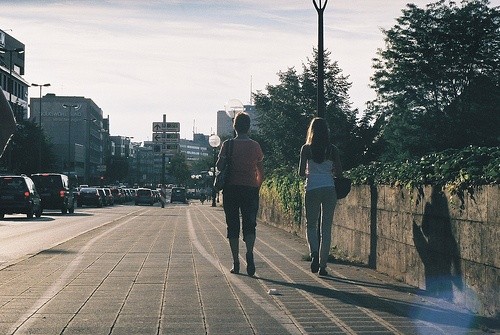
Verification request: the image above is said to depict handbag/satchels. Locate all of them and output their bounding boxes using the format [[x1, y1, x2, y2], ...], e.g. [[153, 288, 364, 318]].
[[211, 138, 233, 191], [333, 178, 351, 198]]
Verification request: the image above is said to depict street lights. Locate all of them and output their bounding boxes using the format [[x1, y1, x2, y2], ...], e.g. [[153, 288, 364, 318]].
[[83, 118, 96, 185], [62, 104, 79, 176], [224, 99, 244, 138], [0, 47, 24, 174], [31, 83, 50, 173], [209, 135, 220, 206]]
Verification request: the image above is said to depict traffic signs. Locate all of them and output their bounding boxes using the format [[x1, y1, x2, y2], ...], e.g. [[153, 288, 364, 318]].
[[153, 122, 180, 132], [153, 144, 180, 154], [153, 133, 180, 143]]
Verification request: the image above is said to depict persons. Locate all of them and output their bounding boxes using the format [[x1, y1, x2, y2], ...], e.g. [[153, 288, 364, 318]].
[[199, 186, 206, 202], [298, 117, 343, 276], [216, 113, 265, 277], [206, 185, 212, 202]]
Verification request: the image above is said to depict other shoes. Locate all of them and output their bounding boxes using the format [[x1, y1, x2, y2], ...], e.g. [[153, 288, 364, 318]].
[[319, 267, 330, 277], [309, 256, 319, 274], [229, 268, 240, 275], [246, 252, 256, 276]]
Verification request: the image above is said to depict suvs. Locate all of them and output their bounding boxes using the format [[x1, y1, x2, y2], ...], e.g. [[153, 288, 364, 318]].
[[0, 174, 42, 219], [169, 187, 188, 203], [29, 173, 75, 214]]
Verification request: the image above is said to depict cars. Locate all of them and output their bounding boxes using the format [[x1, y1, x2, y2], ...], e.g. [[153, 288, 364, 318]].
[[77, 188, 103, 207], [134, 189, 154, 206], [73, 183, 174, 205]]
